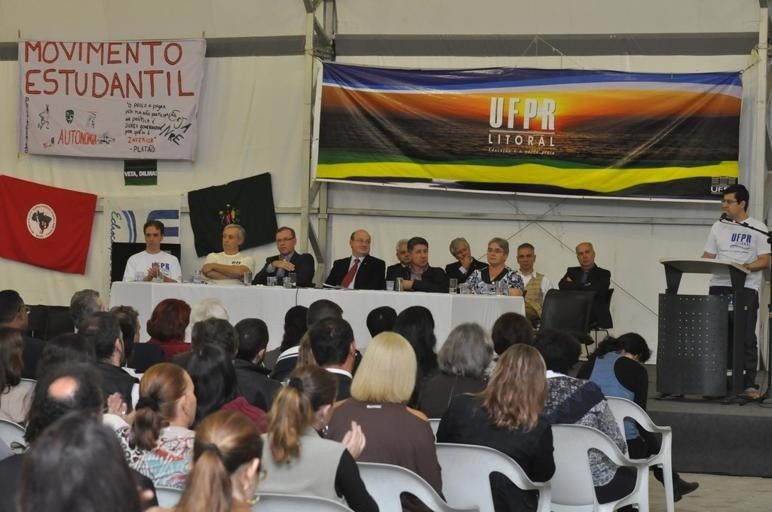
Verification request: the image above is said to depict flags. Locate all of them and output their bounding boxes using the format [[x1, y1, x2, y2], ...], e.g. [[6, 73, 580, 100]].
[[0, 175, 97, 277], [16, 36, 207, 165]]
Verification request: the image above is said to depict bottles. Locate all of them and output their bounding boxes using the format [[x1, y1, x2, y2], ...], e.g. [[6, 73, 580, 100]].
[[218, 203, 241, 231]]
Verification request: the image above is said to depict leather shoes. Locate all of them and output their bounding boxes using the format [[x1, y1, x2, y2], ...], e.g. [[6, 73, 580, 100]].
[[673, 476, 698, 502]]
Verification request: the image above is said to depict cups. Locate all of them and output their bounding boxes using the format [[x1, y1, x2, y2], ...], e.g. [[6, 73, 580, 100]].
[[128, 265, 510, 298]]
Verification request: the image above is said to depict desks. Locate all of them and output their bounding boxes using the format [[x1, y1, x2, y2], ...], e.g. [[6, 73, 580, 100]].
[[108, 279, 526, 357]]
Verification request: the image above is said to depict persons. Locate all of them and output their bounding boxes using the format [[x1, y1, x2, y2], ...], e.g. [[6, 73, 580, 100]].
[[459, 237, 525, 297], [199, 224, 256, 285], [251, 227, 316, 287], [559, 241, 612, 329], [121, 217, 184, 285], [1, 290, 701, 512], [702, 183, 770, 401], [387, 239, 412, 280], [446, 237, 489, 284], [515, 243, 555, 321], [323, 229, 387, 291], [388, 237, 450, 292]]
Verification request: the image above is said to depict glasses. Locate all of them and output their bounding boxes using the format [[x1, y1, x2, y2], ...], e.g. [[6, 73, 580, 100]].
[[18, 306, 31, 314]]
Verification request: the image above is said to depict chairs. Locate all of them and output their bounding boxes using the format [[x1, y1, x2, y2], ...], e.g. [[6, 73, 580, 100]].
[[435, 442, 550, 511], [597, 287, 612, 352], [427, 419, 441, 438], [550, 423, 649, 512], [530, 289, 593, 359], [354, 460, 481, 512], [252, 491, 355, 512], [19, 377, 39, 393], [604, 396, 675, 511], [151, 486, 185, 511]]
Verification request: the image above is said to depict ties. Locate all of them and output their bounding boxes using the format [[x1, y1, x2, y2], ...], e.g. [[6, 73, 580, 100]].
[[340, 259, 360, 289]]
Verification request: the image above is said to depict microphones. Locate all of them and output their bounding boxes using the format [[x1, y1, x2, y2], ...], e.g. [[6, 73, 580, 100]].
[[719, 210, 728, 221]]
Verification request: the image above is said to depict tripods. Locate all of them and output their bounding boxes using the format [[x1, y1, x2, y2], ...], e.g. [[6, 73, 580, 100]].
[[731, 220, 772, 406]]
